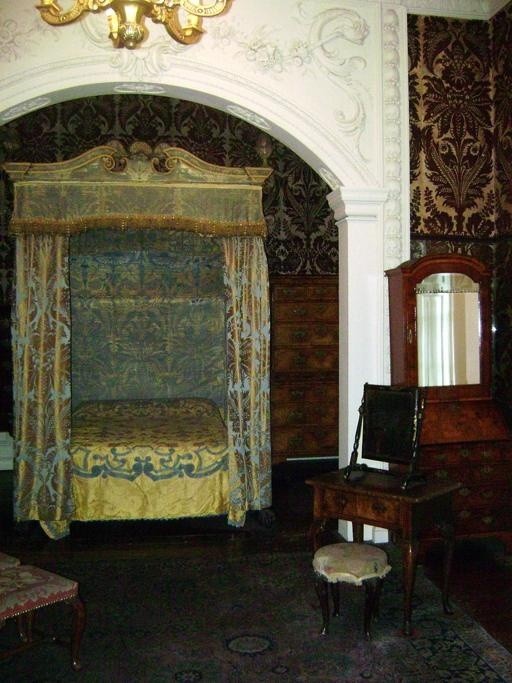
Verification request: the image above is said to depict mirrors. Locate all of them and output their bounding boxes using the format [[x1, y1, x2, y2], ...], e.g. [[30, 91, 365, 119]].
[[400, 254, 494, 404]]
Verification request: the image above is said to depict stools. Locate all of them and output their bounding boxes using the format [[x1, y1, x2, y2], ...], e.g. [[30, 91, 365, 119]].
[[310, 541, 397, 640], [0, 552, 91, 683]]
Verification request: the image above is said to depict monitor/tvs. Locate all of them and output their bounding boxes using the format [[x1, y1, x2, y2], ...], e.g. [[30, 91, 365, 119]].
[[361, 382, 424, 465]]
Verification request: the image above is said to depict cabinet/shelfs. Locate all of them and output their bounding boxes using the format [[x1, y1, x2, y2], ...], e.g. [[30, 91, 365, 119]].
[[382, 253, 512, 557]]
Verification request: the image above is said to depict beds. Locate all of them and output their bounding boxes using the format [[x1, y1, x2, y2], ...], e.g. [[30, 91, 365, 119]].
[[50, 396, 239, 544]]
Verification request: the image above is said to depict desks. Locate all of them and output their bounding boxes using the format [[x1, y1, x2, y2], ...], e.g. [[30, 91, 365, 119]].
[[303, 468, 464, 637]]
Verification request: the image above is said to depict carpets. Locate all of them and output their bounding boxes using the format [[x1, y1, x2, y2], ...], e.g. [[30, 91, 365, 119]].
[[0, 537, 512, 681]]
